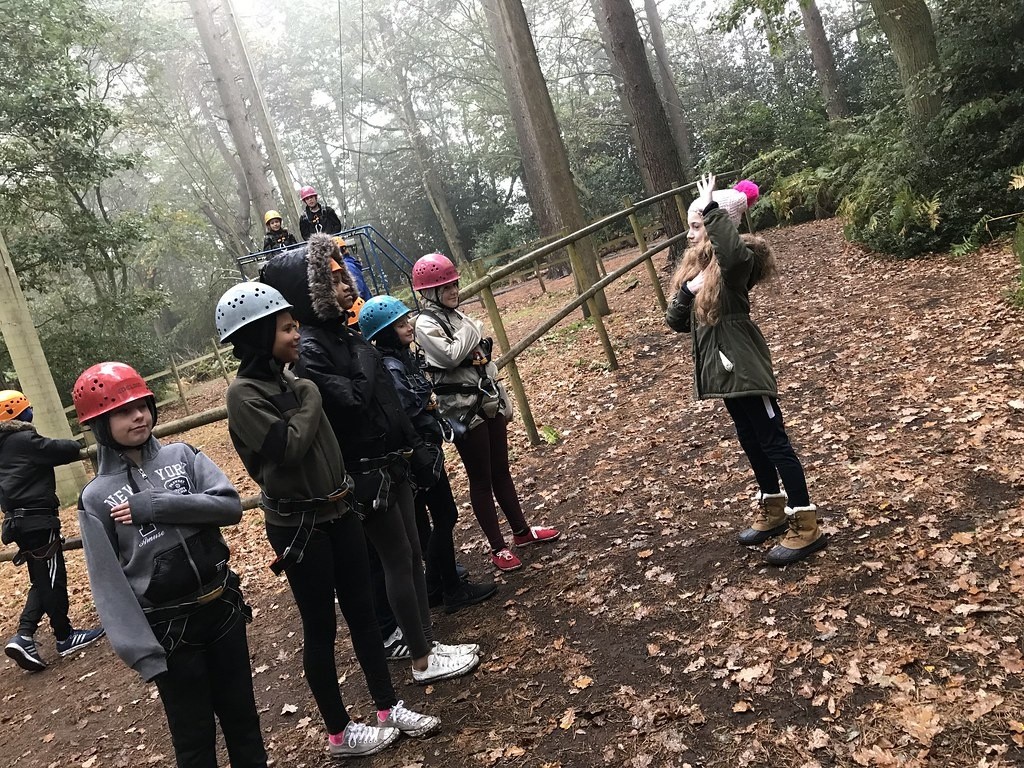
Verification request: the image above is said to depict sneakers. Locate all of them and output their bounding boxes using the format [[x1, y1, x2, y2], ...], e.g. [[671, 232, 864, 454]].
[[5, 635, 46, 670], [412, 648, 479, 684], [513, 527, 560, 548], [329, 719, 400, 757], [428, 583, 444, 608], [431, 640, 480, 655], [381, 627, 410, 660], [56, 628, 105, 657], [443, 576, 497, 613], [377, 700, 439, 736], [492, 546, 522, 571]]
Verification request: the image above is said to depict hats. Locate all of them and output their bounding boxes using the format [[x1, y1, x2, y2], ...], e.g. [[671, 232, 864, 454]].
[[689, 179, 759, 234]]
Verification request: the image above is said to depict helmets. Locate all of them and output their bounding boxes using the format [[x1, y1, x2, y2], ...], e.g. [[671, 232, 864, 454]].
[[331, 257, 344, 273], [332, 236, 346, 248], [301, 186, 317, 200], [412, 254, 460, 291], [358, 295, 411, 341], [73, 362, 154, 424], [347, 296, 365, 326], [215, 282, 293, 342], [0, 390, 31, 420], [265, 210, 282, 224]]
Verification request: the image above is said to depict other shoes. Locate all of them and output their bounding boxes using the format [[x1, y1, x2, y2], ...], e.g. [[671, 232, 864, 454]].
[[455, 566, 469, 578]]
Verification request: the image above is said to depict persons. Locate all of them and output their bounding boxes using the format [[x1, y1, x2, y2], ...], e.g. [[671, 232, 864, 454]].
[[333, 235, 372, 300], [665, 173, 825, 562], [413, 254, 560, 572], [356, 292, 500, 614], [299, 184, 342, 242], [0, 389, 105, 673], [214, 283, 441, 759], [262, 209, 299, 259], [71, 363, 268, 768], [261, 233, 479, 683]]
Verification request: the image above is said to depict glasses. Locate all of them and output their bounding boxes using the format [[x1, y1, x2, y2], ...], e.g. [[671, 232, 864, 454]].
[[28, 406, 33, 412]]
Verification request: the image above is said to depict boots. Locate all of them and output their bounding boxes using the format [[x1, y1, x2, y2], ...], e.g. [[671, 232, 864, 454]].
[[766, 503, 828, 565], [737, 489, 789, 545]]
